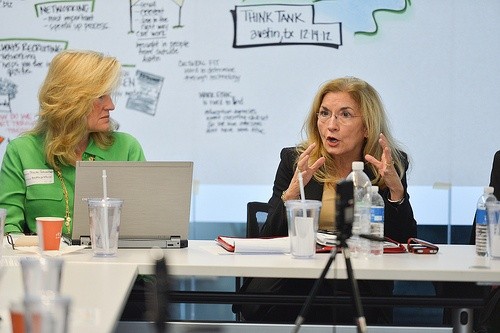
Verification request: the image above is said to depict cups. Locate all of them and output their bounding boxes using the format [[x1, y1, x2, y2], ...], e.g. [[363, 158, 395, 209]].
[[19, 256, 65, 295], [86, 198, 123, 253], [0, 208, 7, 249], [285, 199, 321, 258], [485, 200, 500, 261], [27, 294, 72, 332], [36, 217, 65, 254], [9, 300, 26, 333]]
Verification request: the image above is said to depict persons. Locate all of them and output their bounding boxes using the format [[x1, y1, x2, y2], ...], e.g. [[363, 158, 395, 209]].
[[231, 75, 420, 324], [442, 150, 500, 332], [0, 50, 180, 323]]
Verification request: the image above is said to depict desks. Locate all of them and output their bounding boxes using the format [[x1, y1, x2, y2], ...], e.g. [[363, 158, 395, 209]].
[[0, 237, 500, 333]]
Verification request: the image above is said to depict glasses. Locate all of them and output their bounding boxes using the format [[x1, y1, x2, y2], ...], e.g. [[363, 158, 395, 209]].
[[313, 110, 363, 120]]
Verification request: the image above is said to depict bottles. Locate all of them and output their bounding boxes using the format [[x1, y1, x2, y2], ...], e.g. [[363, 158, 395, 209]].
[[346, 162, 372, 260], [475, 186, 497, 256], [372, 186, 384, 256]]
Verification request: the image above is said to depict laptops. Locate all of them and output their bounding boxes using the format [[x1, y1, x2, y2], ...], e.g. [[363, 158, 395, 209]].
[[60, 161, 192, 249]]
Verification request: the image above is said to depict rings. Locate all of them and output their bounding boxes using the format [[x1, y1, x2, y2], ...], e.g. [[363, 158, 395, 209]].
[[385, 161, 394, 167], [298, 167, 303, 170]]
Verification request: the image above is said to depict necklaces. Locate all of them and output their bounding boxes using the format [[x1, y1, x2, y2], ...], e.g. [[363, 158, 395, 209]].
[[54, 156, 96, 236]]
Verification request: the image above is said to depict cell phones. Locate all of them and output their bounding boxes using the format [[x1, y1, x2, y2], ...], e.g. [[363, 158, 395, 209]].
[[408, 239, 439, 255]]
